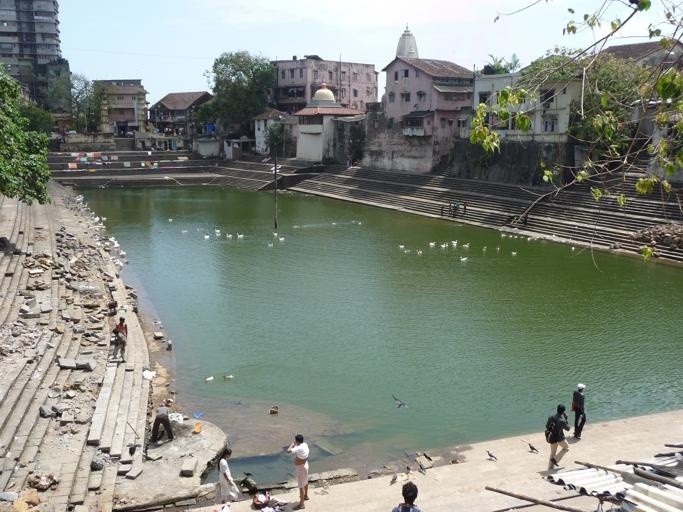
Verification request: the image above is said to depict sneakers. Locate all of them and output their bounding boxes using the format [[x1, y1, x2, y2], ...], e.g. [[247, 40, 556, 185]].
[[574, 432, 581, 439], [551, 458, 559, 467]]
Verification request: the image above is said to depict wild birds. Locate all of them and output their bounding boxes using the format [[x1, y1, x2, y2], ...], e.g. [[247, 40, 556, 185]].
[[204, 229, 244, 240], [486, 451, 497, 460], [268, 232, 285, 248], [270, 406, 278, 414], [398, 232, 577, 263], [392, 393, 409, 409], [205, 375, 213, 381], [391, 471, 397, 481], [77, 193, 128, 270], [351, 218, 362, 225], [223, 375, 234, 379], [528, 443, 539, 453]]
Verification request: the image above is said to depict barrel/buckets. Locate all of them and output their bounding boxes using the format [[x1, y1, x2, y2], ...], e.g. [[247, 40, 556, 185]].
[[194, 422, 203, 432]]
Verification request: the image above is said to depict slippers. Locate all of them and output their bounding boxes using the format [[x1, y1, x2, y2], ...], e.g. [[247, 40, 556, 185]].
[[292, 495, 309, 510]]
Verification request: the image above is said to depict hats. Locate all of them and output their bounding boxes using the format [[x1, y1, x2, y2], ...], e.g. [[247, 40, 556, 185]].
[[578, 384, 585, 389]]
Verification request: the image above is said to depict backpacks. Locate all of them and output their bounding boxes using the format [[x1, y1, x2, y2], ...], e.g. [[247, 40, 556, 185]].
[[545, 416, 560, 443]]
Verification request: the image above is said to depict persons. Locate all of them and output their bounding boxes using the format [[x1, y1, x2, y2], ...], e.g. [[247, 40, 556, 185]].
[[344, 158, 354, 166], [111, 317, 129, 360], [545, 404, 570, 469], [439, 199, 468, 215], [572, 384, 586, 439], [288, 435, 310, 510], [151, 407, 172, 441], [523, 214, 527, 224], [391, 482, 421, 512], [218, 447, 240, 503]]
